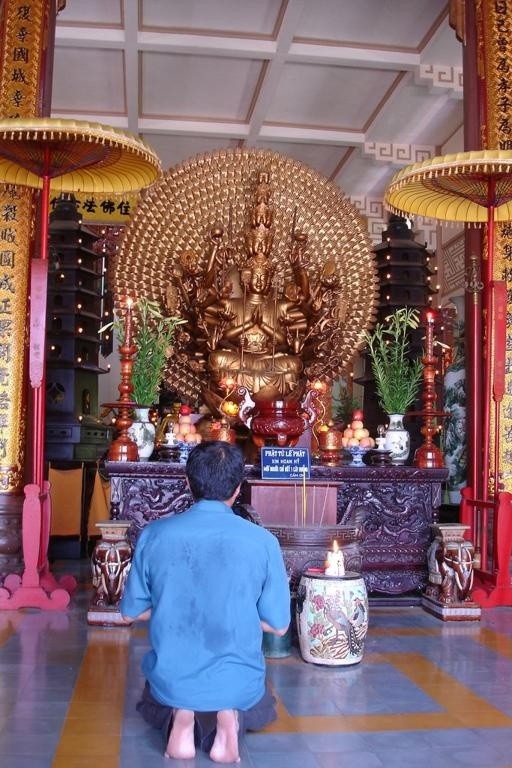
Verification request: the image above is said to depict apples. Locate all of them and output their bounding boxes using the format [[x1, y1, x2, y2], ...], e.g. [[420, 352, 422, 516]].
[[341, 409, 375, 448], [173, 406, 203, 443]]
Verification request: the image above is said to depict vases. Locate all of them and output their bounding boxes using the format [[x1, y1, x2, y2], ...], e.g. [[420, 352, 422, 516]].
[[127, 407, 156, 462], [86, 521, 133, 627], [420, 522, 481, 621], [382, 414, 411, 466]]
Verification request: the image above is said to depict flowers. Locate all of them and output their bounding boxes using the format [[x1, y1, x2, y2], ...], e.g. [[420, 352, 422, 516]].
[[98, 294, 189, 406], [355, 304, 450, 414]]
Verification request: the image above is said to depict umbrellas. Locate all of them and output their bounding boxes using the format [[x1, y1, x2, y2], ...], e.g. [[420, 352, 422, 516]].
[[1, 115, 165, 492], [381, 149, 512, 572]]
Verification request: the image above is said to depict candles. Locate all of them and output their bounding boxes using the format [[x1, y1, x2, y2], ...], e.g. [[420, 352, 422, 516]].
[[425, 312, 434, 363], [123, 298, 132, 348]]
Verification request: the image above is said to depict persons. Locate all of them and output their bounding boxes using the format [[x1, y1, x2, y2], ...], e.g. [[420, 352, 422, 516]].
[[168, 172, 341, 404], [119, 439, 292, 764]]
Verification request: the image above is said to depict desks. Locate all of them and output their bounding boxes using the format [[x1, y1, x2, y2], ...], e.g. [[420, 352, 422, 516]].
[[296, 570, 369, 666]]
